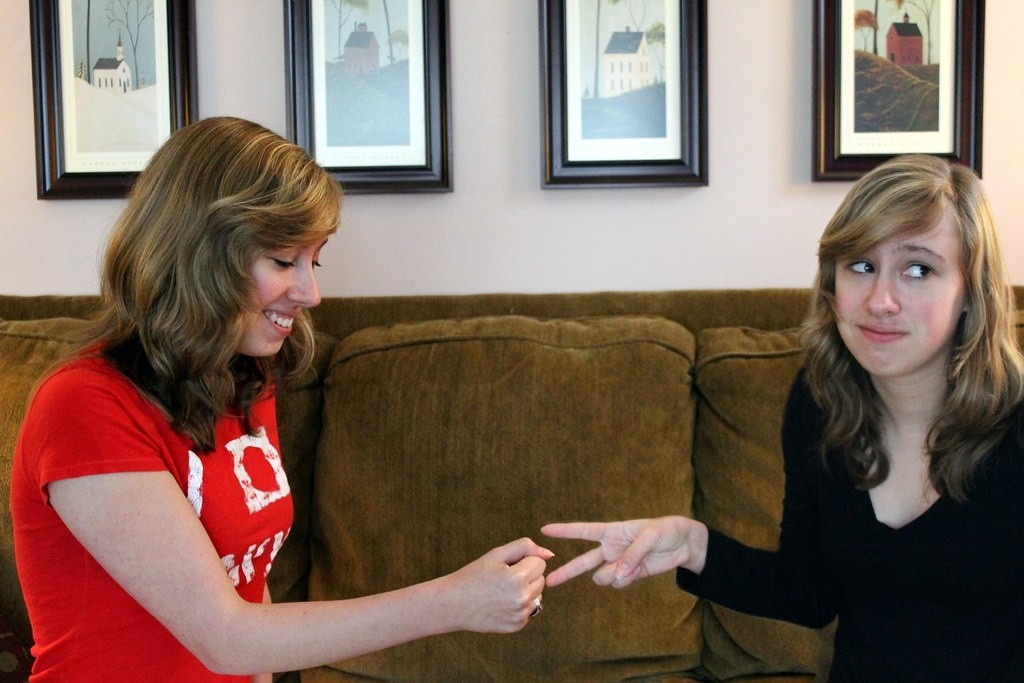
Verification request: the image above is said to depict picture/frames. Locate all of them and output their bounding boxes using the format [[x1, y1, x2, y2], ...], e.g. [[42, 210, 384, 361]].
[[537, 0, 708, 189], [29, 0, 196, 199], [811, 0, 985, 180], [282, 0, 453, 195]]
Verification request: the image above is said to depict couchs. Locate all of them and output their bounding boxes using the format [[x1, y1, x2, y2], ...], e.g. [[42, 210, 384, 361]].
[[0, 285, 1024, 683]]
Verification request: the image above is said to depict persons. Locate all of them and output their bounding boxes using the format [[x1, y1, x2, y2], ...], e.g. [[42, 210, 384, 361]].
[[10, 116, 555, 683], [541, 156, 1024, 683]]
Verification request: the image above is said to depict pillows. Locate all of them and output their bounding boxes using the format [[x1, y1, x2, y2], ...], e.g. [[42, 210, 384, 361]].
[[305, 313, 702, 683], [691, 324, 841, 679], [0, 318, 336, 683]]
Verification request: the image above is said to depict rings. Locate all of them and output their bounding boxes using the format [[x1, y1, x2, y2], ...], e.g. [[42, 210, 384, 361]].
[[531, 599, 541, 618]]
